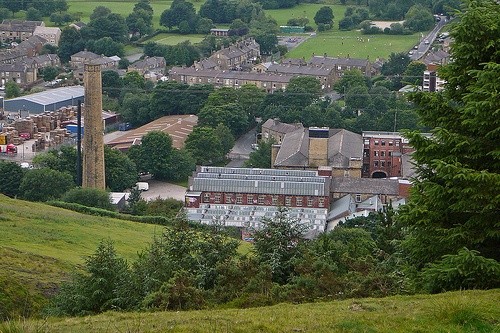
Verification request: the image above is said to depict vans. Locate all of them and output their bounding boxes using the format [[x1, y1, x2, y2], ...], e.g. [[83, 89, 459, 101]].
[[251, 144, 259, 150], [19, 133, 30, 140]]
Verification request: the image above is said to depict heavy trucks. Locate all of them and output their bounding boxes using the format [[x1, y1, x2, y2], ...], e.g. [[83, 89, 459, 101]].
[[0, 144, 17, 157]]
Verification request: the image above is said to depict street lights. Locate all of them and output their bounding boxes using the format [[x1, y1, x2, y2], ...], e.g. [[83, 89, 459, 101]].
[[255, 133, 262, 144]]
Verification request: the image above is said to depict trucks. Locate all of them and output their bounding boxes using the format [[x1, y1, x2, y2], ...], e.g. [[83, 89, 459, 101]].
[[132, 181, 149, 191], [0, 134, 7, 145]]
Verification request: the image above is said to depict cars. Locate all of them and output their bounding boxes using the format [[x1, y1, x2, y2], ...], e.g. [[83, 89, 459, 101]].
[[255, 146, 259, 151], [140, 171, 150, 175]]
